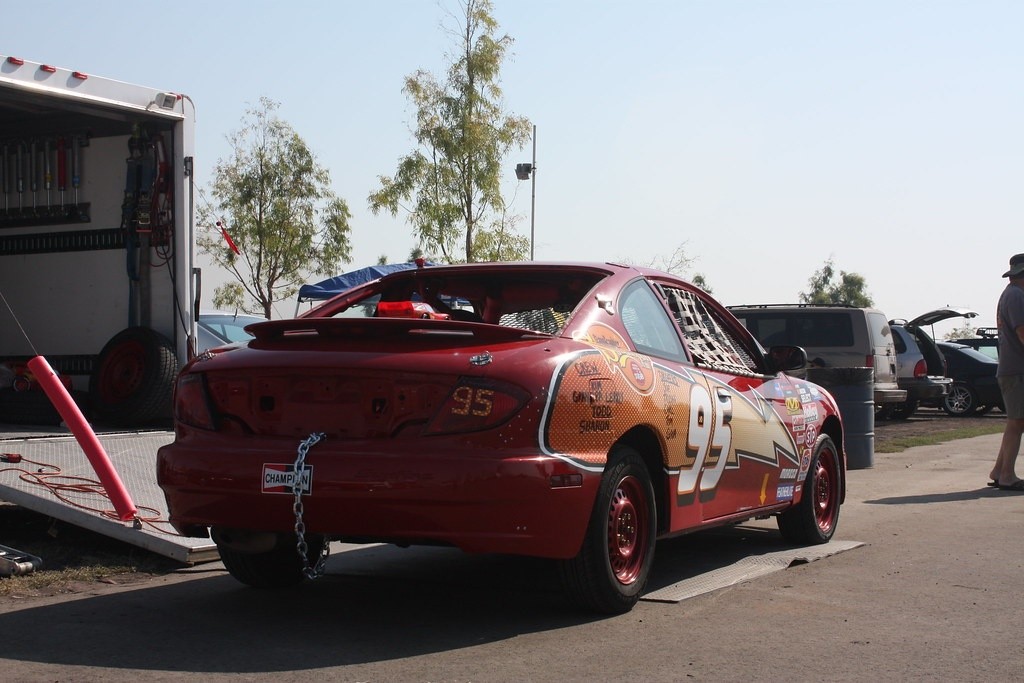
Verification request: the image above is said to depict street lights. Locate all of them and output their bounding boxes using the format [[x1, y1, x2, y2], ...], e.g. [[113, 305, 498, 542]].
[[515, 125, 536, 263]]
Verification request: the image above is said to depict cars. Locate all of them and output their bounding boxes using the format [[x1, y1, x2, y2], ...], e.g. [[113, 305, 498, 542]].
[[933, 338, 999, 418], [156, 258, 845, 616], [197, 311, 270, 355]]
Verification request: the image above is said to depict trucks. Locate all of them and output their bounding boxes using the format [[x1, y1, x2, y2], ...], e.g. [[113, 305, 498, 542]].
[[0, 56, 221, 566]]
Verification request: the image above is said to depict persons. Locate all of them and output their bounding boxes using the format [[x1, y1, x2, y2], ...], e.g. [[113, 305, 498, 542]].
[[987, 254, 1024, 491]]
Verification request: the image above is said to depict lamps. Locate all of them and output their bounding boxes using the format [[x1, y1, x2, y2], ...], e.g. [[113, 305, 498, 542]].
[[146, 92, 176, 111]]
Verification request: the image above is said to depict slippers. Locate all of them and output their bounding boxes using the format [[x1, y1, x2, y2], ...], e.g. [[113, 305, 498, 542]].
[[987, 479, 1024, 490]]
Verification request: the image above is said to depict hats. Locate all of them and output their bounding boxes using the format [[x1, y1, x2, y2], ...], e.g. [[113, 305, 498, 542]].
[[1001, 253, 1024, 278]]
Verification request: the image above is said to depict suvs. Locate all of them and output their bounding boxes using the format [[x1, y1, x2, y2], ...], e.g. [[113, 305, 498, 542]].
[[945, 326, 998, 363], [722, 303, 908, 424], [887, 304, 979, 420]]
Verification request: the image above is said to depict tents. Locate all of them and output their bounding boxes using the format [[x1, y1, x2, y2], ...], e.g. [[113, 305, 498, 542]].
[[294, 261, 471, 317]]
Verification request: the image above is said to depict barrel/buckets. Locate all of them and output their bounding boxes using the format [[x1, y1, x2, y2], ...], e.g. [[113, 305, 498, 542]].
[[805, 367, 875, 467]]
